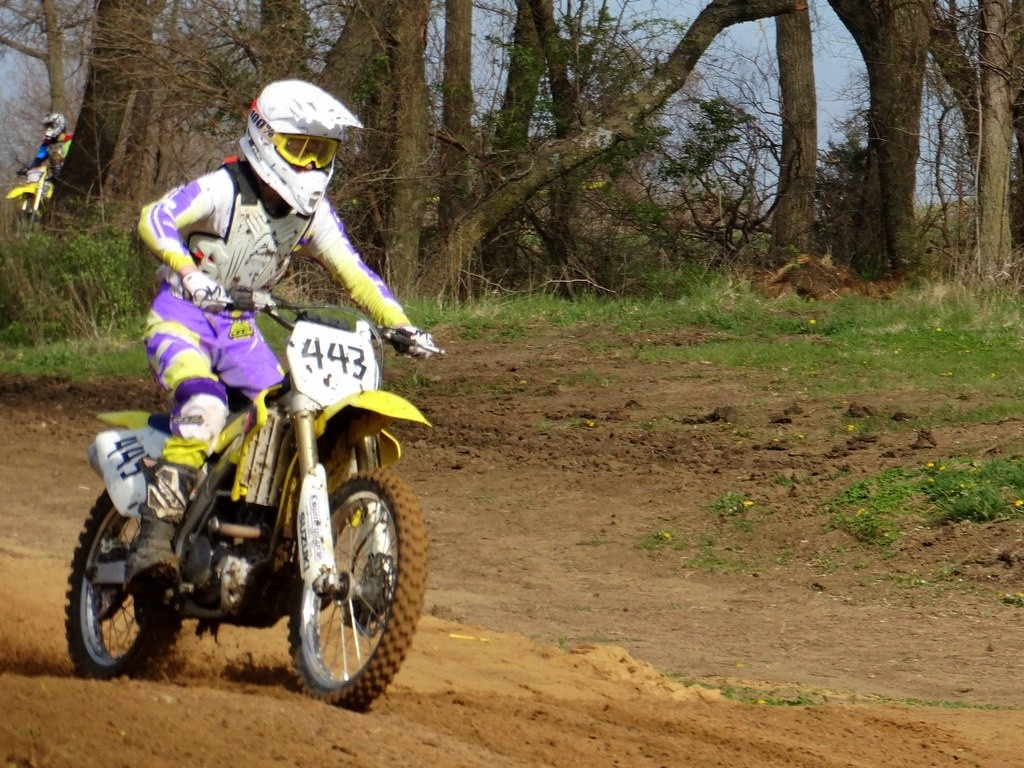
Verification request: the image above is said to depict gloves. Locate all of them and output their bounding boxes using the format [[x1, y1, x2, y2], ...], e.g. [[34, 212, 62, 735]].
[[392, 324, 435, 361], [17, 167, 29, 175], [50, 164, 62, 177], [182, 272, 227, 314]]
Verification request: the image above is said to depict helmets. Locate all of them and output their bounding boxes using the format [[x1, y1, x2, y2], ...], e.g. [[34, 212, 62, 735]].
[[41, 113, 67, 140], [238, 78, 364, 216]]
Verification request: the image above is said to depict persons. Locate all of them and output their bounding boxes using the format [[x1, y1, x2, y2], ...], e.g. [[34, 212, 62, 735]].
[[124, 79, 437, 591], [15, 113, 76, 176]]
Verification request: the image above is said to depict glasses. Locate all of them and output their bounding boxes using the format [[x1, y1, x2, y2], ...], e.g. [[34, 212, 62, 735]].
[[248, 98, 338, 169]]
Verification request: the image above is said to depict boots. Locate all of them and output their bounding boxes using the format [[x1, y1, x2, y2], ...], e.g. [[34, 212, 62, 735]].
[[123, 503, 181, 597]]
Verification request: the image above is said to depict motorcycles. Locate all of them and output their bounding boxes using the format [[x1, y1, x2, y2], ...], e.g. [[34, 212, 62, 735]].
[[61, 288, 449, 718], [4, 138, 59, 234]]
